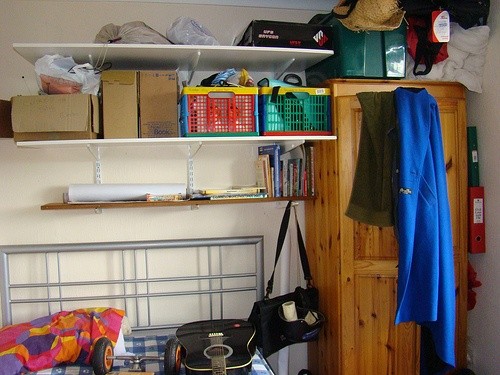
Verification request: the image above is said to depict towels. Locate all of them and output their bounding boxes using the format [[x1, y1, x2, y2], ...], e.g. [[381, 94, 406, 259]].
[[395, 87, 456, 375], [345, 91, 397, 227]]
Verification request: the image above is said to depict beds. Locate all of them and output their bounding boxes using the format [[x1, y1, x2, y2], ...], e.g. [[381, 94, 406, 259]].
[[0, 235, 278, 375]]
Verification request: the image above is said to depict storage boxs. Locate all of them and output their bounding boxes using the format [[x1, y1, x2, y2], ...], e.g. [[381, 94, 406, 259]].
[[232, 12, 407, 79], [11, 94, 99, 141], [100, 70, 139, 139], [140, 70, 178, 138], [467, 127, 487, 254]]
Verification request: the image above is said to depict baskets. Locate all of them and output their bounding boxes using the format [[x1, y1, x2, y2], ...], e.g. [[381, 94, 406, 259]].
[[180, 86, 259, 137], [259, 85, 332, 136]]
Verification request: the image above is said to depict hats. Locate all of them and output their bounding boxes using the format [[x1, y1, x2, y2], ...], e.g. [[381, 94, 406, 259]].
[[332, 1, 406, 31]]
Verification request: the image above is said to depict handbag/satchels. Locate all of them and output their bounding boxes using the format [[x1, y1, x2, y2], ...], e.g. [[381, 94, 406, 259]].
[[251, 286, 324, 355], [400, 0, 448, 75], [35, 54, 103, 95], [438, 0, 490, 30]]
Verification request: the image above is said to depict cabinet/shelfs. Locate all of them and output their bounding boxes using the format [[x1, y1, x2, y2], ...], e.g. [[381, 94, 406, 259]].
[[307, 80, 468, 375], [10, 41, 336, 209]]
